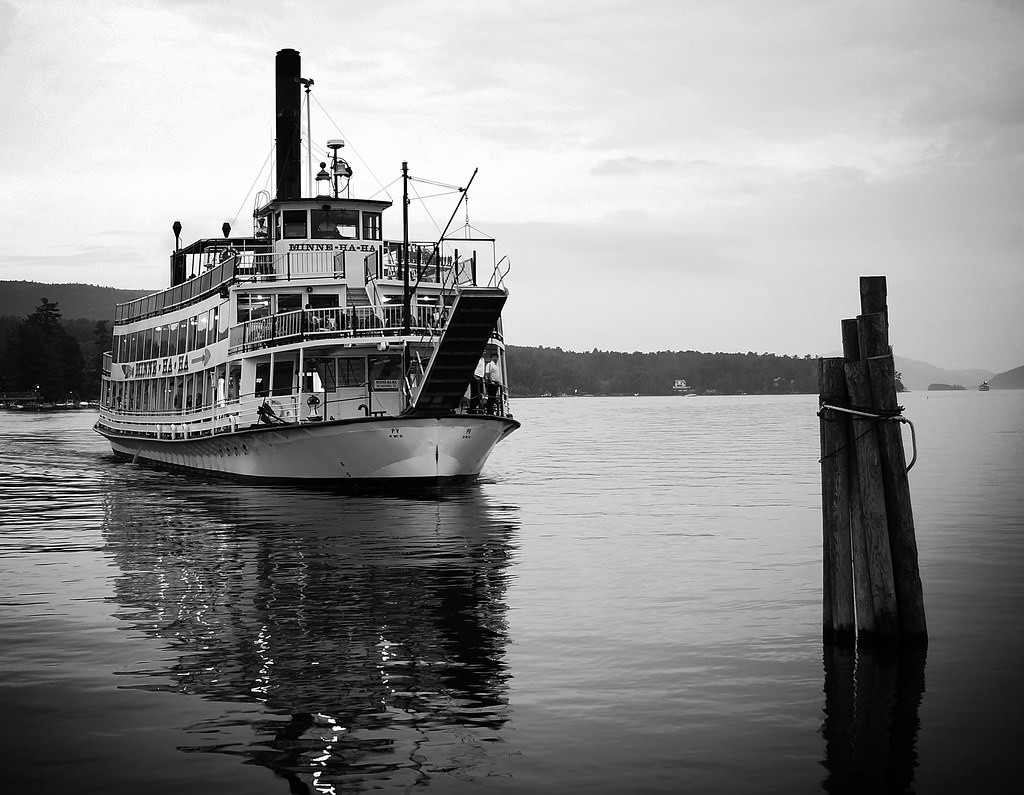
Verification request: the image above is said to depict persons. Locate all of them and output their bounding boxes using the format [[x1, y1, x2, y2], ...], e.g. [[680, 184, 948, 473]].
[[466, 348, 486, 414], [486, 352, 500, 415], [317, 212, 338, 238]]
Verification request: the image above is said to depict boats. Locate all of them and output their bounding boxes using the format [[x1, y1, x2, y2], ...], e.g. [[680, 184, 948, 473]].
[[92, 47, 522, 483]]
